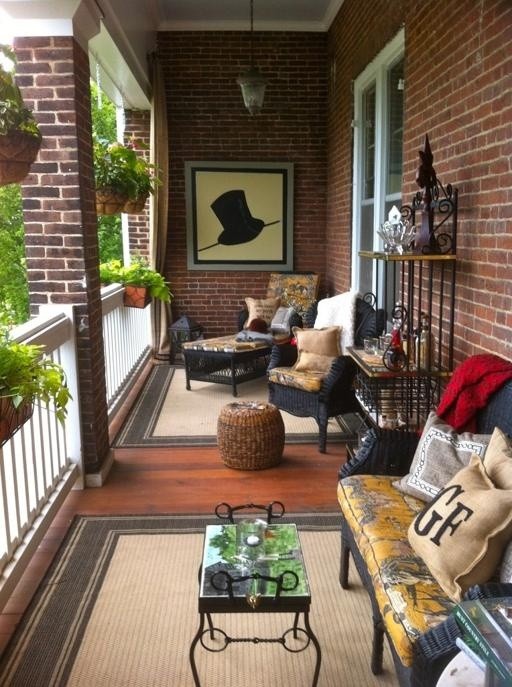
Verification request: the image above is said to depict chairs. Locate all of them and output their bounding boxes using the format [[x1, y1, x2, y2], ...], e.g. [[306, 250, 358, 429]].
[[181, 271, 319, 396], [265, 294, 383, 455]]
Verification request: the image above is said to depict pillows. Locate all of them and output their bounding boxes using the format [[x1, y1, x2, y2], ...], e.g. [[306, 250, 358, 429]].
[[407, 458, 512, 599], [481, 427, 512, 487], [392, 413, 488, 500], [242, 289, 363, 372]]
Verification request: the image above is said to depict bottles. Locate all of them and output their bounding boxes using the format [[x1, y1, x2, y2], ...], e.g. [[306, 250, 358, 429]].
[[378, 302, 434, 370]]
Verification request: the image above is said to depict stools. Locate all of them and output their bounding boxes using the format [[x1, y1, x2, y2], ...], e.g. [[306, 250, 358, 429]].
[[216, 400, 284, 470]]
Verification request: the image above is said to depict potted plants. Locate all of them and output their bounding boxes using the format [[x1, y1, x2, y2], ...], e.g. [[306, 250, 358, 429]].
[[121, 258, 175, 309], [90, 137, 128, 215], [0, 332, 72, 450], [122, 146, 165, 214], [0, 42, 41, 186]]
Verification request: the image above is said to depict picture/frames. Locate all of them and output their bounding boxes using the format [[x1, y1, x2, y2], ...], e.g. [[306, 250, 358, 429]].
[[185, 158, 295, 272]]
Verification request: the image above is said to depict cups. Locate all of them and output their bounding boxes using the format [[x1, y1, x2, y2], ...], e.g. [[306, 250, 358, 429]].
[[364, 336, 378, 355], [234, 518, 266, 559]]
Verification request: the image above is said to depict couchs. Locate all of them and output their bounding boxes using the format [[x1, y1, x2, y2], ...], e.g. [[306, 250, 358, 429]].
[[337, 353, 512, 687]]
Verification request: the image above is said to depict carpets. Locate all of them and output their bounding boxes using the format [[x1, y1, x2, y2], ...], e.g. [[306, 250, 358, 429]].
[[109, 360, 372, 447], [0, 512, 413, 687]]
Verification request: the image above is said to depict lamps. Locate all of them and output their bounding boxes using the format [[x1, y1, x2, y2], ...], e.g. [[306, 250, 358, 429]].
[[234, 0, 268, 116]]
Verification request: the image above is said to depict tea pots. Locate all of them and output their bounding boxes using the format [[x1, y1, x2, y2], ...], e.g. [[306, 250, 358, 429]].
[[377, 220, 416, 254]]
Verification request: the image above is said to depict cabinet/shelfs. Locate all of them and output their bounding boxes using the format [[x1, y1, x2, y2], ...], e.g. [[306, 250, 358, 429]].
[[345, 134, 458, 460]]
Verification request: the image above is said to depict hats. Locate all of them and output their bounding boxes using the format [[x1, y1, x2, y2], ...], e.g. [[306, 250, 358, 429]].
[[210, 191, 264, 246]]
